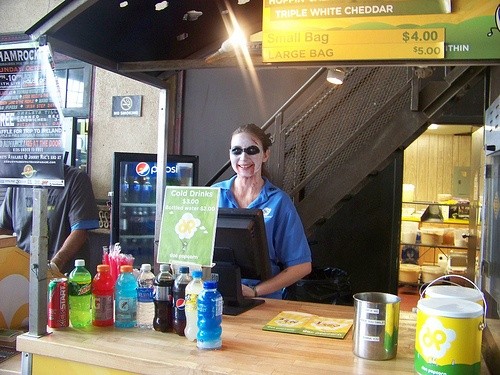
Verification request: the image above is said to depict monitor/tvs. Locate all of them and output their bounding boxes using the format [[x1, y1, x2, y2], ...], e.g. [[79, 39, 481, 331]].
[[211, 208, 272, 316]]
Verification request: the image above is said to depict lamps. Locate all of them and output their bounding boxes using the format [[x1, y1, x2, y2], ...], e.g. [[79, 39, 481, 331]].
[[327, 67, 346, 84]]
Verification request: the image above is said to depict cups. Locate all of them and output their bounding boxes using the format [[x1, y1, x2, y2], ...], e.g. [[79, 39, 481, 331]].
[[352, 292, 401, 360]]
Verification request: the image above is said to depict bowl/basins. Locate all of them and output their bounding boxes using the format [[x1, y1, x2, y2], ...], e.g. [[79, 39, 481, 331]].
[[402, 184, 415, 202], [401, 222, 482, 248], [398, 266, 467, 286]]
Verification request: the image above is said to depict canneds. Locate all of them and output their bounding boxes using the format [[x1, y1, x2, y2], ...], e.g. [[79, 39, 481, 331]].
[[48, 277, 69, 328]]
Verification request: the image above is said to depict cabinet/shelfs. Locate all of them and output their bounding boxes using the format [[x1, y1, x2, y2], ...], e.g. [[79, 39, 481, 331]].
[[399, 242, 480, 288]]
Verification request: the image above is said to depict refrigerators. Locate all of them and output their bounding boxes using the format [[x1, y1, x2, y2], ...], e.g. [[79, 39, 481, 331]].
[[110, 153, 200, 274]]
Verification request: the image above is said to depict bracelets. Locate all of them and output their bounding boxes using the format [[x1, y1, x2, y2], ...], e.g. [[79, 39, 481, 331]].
[[254, 287, 257, 297]]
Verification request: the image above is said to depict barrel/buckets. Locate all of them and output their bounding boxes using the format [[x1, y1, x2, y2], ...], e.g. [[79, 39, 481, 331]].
[[413, 274, 486, 375]]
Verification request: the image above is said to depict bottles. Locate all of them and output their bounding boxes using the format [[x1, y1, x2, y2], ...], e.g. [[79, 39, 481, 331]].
[[121, 175, 156, 265], [172, 266, 192, 336], [152, 265, 175, 333], [196, 280, 223, 350], [68, 259, 91, 329], [183, 270, 204, 341], [114, 265, 138, 328], [136, 264, 156, 329], [91, 265, 114, 327]]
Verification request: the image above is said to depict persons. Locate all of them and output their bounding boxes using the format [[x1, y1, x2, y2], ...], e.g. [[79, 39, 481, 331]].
[[208, 123, 313, 300], [0, 164, 99, 279]]
